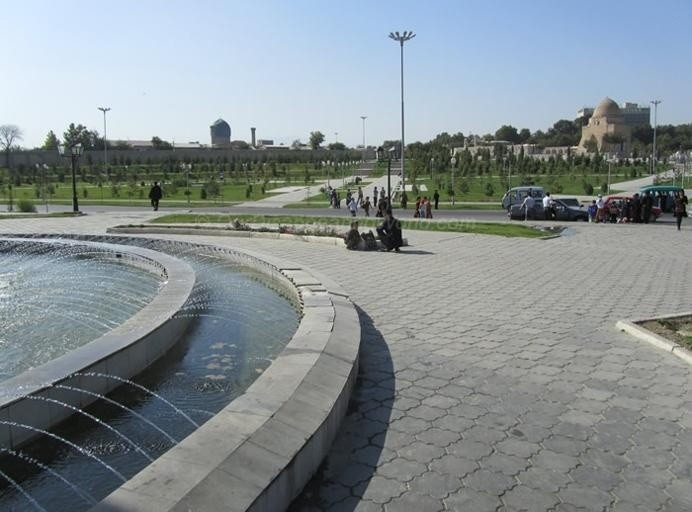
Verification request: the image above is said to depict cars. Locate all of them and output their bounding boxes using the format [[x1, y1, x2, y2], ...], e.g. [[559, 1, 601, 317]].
[[596, 195, 664, 222], [508, 199, 588, 221]]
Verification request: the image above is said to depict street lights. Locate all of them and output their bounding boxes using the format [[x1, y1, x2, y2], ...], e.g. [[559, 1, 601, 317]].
[[606, 159, 615, 195], [360, 116, 368, 149], [180, 162, 192, 212], [676, 150, 692, 185], [98, 107, 111, 186], [651, 101, 661, 173], [338, 160, 365, 192], [58, 142, 84, 212], [430, 157, 434, 181], [35, 162, 49, 201], [388, 31, 416, 189], [451, 158, 456, 207], [322, 160, 334, 192]]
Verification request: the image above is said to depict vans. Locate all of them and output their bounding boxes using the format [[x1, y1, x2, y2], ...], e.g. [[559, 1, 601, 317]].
[[501, 186, 544, 209], [639, 184, 684, 214]]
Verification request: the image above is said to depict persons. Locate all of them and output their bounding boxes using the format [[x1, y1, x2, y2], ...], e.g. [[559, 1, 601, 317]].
[[149, 181, 162, 211], [343, 221, 360, 249], [375, 209, 403, 252], [596, 194, 606, 223], [401, 191, 407, 208], [329, 187, 391, 217], [672, 189, 688, 230], [520, 192, 535, 220], [542, 192, 556, 220], [610, 191, 652, 223], [587, 200, 598, 222], [434, 190, 439, 209], [414, 196, 432, 218]]
